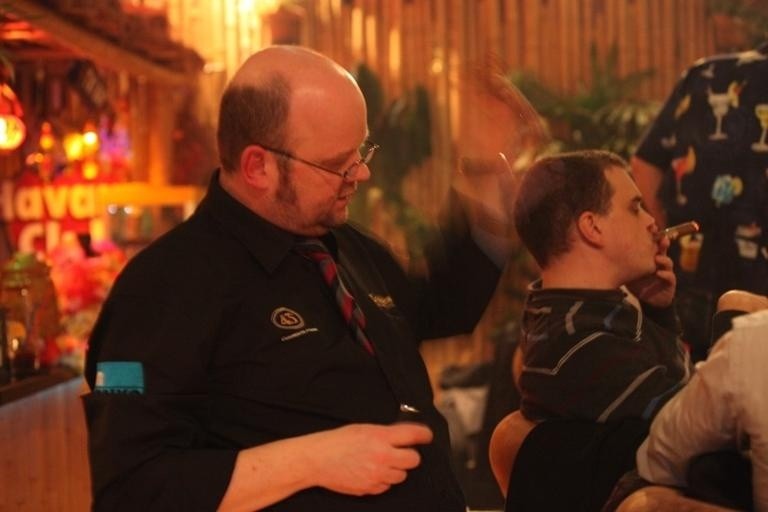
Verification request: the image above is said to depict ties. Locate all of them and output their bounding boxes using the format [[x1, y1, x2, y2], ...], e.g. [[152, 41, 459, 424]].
[[291, 236, 376, 357]]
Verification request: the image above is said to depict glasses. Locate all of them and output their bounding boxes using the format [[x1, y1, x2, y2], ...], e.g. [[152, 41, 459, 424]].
[[257, 141, 378, 182]]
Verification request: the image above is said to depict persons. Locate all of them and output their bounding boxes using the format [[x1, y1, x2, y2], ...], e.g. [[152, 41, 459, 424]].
[[82, 45, 463, 511]]
